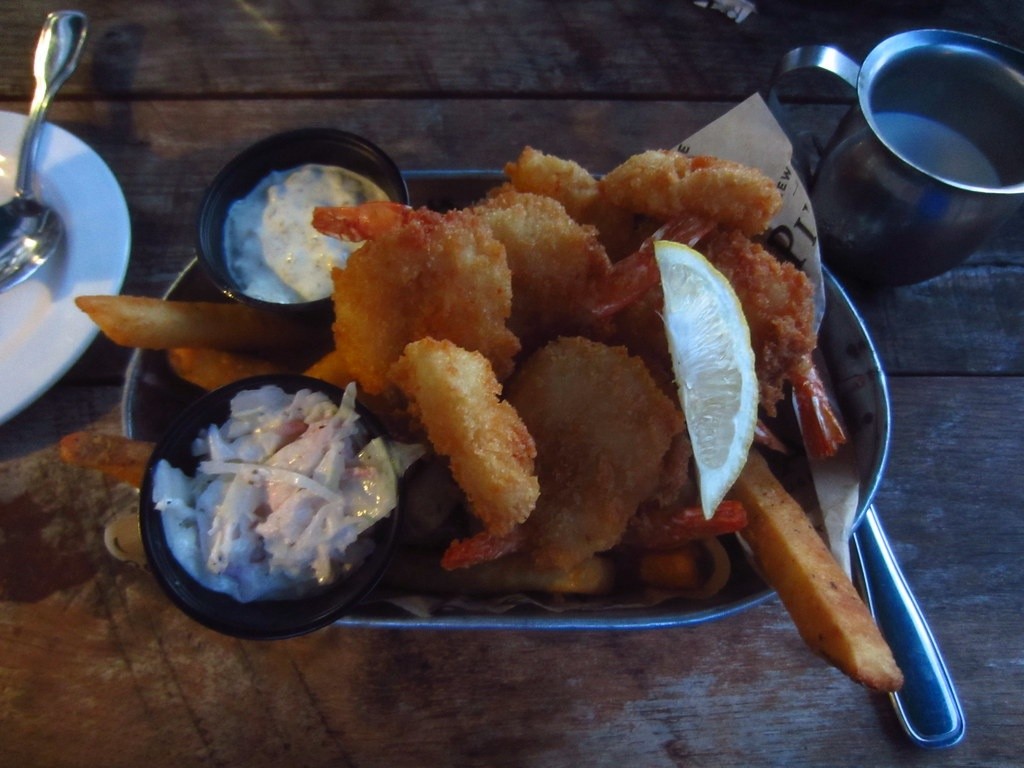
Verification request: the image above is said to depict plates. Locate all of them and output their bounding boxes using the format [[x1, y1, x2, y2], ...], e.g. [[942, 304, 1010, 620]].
[[126, 170, 893, 631], [0, 111, 132, 426]]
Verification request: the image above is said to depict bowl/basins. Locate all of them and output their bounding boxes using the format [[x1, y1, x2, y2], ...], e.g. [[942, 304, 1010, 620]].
[[197, 129, 410, 327], [137, 374, 398, 640]]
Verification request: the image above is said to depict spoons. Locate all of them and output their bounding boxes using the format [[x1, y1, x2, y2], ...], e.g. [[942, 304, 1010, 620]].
[[0, 12, 88, 294]]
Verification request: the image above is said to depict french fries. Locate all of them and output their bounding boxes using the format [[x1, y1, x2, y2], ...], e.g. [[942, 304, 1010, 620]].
[[58, 294, 333, 488], [723, 450, 903, 691]]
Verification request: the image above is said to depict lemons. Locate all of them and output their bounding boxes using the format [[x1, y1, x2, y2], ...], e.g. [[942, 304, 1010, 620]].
[[655, 239, 759, 519]]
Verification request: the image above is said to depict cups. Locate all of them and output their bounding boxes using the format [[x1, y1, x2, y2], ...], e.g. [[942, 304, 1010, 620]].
[[785, 30, 1024, 301]]
